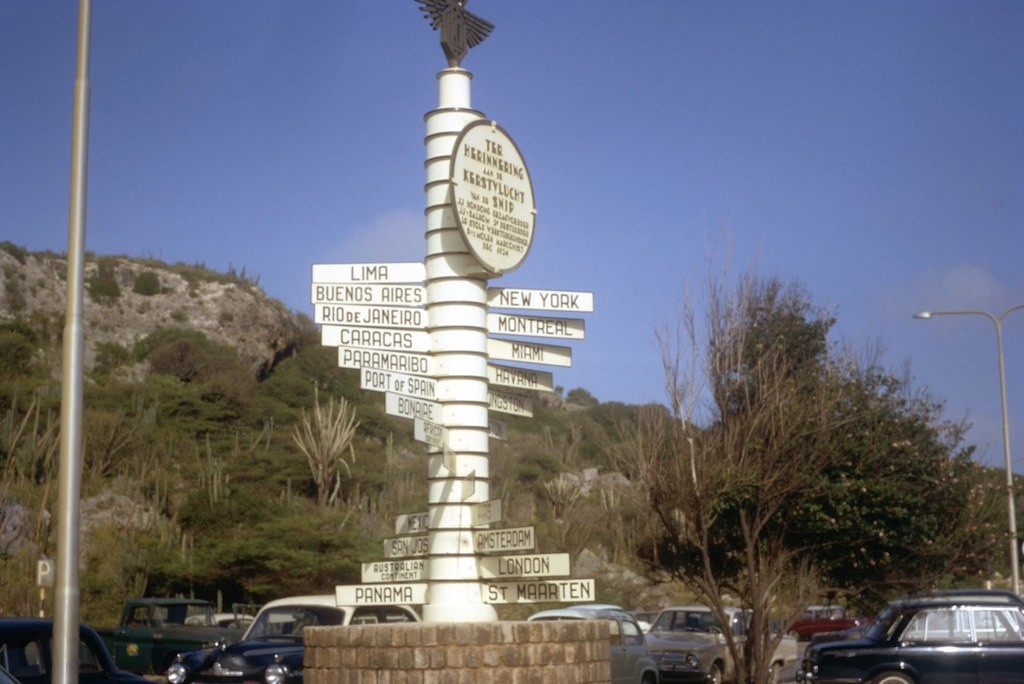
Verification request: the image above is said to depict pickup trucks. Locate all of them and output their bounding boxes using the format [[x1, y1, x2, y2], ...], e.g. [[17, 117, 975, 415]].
[[1, 598, 421, 684], [527, 603, 799, 683]]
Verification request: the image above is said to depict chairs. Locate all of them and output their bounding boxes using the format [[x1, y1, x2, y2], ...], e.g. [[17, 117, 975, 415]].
[[687, 617, 699, 629]]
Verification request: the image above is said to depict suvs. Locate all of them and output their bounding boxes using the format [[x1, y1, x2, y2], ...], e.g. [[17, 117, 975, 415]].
[[768, 589, 1024, 683]]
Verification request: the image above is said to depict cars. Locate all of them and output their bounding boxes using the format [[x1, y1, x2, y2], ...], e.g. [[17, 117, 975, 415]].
[[167, 595, 422, 684]]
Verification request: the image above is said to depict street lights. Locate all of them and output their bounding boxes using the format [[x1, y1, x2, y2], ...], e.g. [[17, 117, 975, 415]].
[[912, 305, 1024, 596]]
